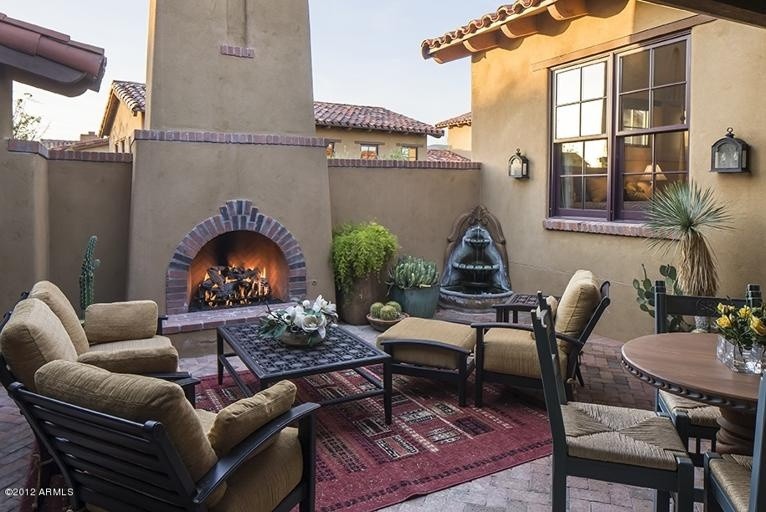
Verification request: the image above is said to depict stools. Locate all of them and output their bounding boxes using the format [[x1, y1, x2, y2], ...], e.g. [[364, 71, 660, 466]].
[[377, 317, 487, 408]]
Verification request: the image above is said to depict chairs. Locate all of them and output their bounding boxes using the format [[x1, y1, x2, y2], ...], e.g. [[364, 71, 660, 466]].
[[8, 360, 321, 512]]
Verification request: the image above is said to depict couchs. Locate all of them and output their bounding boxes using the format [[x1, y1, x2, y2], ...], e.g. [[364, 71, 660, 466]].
[[0, 292, 190, 512]]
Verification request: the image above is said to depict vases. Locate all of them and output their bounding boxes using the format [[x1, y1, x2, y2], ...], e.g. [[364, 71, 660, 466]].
[[280, 333, 320, 346]]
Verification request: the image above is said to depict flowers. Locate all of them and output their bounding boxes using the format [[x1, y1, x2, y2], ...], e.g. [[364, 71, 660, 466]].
[[252, 294, 339, 342]]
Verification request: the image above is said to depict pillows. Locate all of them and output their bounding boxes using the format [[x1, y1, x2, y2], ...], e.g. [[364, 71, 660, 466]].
[[208, 380, 297, 460], [34, 359, 227, 508], [78, 346, 179, 374], [0, 299, 77, 394], [28, 280, 90, 355], [84, 299, 158, 342]]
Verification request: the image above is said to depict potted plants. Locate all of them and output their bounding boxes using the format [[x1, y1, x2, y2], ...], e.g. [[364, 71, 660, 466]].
[[330, 221, 440, 332]]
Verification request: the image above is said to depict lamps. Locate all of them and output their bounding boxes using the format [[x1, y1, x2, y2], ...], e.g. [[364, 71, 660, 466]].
[[509, 148, 529, 179], [710, 127, 751, 173]]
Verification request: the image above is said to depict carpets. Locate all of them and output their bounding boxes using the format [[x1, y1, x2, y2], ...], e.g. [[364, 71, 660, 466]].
[[19, 361, 553, 512]]
